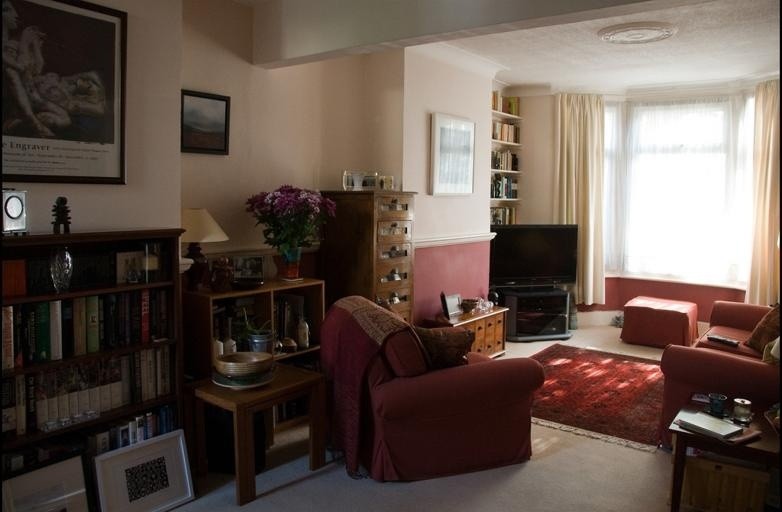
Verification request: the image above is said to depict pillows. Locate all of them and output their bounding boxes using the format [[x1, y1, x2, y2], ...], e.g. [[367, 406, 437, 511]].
[[407, 322, 479, 370], [745, 302, 782, 353]]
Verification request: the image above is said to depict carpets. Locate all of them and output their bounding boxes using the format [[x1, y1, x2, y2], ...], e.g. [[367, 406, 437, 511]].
[[527, 343, 664, 453]]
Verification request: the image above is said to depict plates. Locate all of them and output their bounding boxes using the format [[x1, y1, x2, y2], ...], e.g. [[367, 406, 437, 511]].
[[213, 372, 275, 392], [229, 278, 266, 289]]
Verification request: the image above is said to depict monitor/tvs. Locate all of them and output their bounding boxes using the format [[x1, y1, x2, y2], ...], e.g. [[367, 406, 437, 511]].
[[489, 224, 579, 285]]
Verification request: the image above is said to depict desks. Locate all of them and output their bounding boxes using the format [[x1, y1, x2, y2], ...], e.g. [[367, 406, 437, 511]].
[[187, 366, 328, 507], [666, 391, 782, 511]]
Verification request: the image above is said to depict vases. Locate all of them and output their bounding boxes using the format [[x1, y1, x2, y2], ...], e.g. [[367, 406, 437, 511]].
[[274, 255, 301, 280]]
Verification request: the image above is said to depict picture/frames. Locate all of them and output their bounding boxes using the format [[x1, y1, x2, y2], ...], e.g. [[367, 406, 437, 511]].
[[430, 111, 477, 198], [178, 85, 232, 156], [447, 292, 463, 318], [341, 170, 379, 192], [109, 244, 151, 286], [232, 255, 264, 287], [94, 429, 196, 512], [0, 0, 129, 184], [1, 450, 95, 511]]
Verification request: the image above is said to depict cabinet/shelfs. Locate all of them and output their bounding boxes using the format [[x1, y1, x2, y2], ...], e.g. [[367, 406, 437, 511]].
[[316, 184, 420, 328], [1, 229, 185, 481], [490, 285, 577, 343], [491, 108, 523, 223], [424, 301, 510, 359], [183, 276, 328, 431]]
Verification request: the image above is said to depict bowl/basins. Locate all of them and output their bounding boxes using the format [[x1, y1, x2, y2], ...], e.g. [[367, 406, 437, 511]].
[[213, 352, 274, 381]]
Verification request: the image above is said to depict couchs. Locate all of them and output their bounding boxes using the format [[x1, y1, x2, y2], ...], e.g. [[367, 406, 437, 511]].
[[656, 292, 781, 450], [317, 293, 546, 482], [621, 291, 700, 347]]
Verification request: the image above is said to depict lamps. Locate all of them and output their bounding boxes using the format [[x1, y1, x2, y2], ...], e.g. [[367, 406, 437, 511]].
[[181, 207, 230, 279], [596, 22, 680, 46], [3, 186, 30, 235]]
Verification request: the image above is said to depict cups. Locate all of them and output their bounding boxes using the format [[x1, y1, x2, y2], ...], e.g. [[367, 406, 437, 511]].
[[139, 242, 161, 282], [733, 397, 753, 421], [709, 393, 727, 412], [341, 168, 396, 192]]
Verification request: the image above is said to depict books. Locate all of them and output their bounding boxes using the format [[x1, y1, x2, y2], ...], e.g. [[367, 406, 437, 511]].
[[676, 409, 762, 445], [677, 412, 744, 440], [0, 285, 178, 478]]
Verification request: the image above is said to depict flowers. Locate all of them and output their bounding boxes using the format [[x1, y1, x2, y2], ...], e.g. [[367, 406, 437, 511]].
[[246, 175, 336, 263]]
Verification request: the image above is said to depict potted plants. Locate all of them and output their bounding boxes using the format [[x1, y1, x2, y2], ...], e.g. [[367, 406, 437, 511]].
[[240, 306, 276, 355]]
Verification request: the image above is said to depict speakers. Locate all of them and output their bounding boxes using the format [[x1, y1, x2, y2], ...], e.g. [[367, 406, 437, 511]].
[[0, 189, 29, 236]]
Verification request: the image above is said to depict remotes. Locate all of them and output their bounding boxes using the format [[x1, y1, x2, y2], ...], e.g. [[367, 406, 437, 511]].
[[707, 335, 738, 347]]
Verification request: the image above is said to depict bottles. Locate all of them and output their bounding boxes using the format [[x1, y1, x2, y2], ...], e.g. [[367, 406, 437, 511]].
[[298, 320, 309, 348]]
